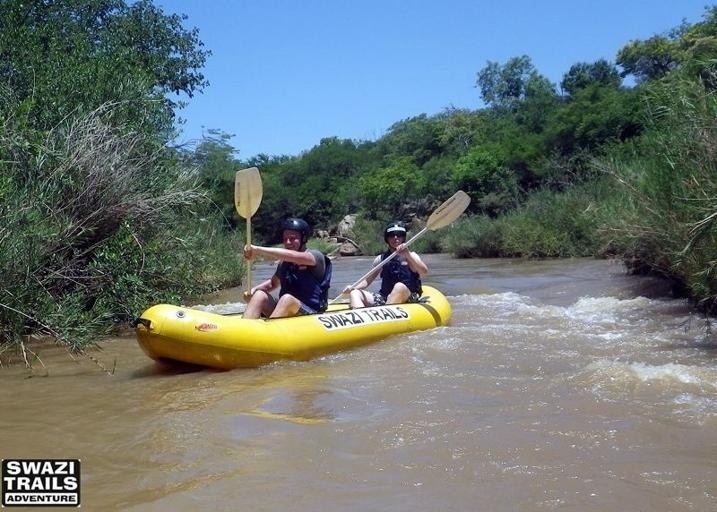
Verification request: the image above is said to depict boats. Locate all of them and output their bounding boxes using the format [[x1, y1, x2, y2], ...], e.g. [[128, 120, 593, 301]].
[[134, 285, 452, 371]]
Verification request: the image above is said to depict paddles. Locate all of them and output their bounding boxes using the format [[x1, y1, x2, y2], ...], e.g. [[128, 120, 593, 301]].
[[234, 167, 263, 296], [334, 190, 470, 303]]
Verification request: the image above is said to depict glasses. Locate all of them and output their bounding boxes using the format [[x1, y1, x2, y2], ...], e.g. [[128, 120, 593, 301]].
[[389, 232, 404, 236]]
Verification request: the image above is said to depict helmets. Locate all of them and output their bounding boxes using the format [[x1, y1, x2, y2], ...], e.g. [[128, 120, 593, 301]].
[[284, 218, 309, 235]]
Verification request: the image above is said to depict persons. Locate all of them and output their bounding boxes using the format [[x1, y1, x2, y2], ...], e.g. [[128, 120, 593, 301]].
[[342, 222, 428, 310], [241, 217, 332, 320]]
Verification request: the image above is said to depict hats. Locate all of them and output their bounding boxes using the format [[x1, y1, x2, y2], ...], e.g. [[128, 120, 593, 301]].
[[387, 222, 406, 232]]
